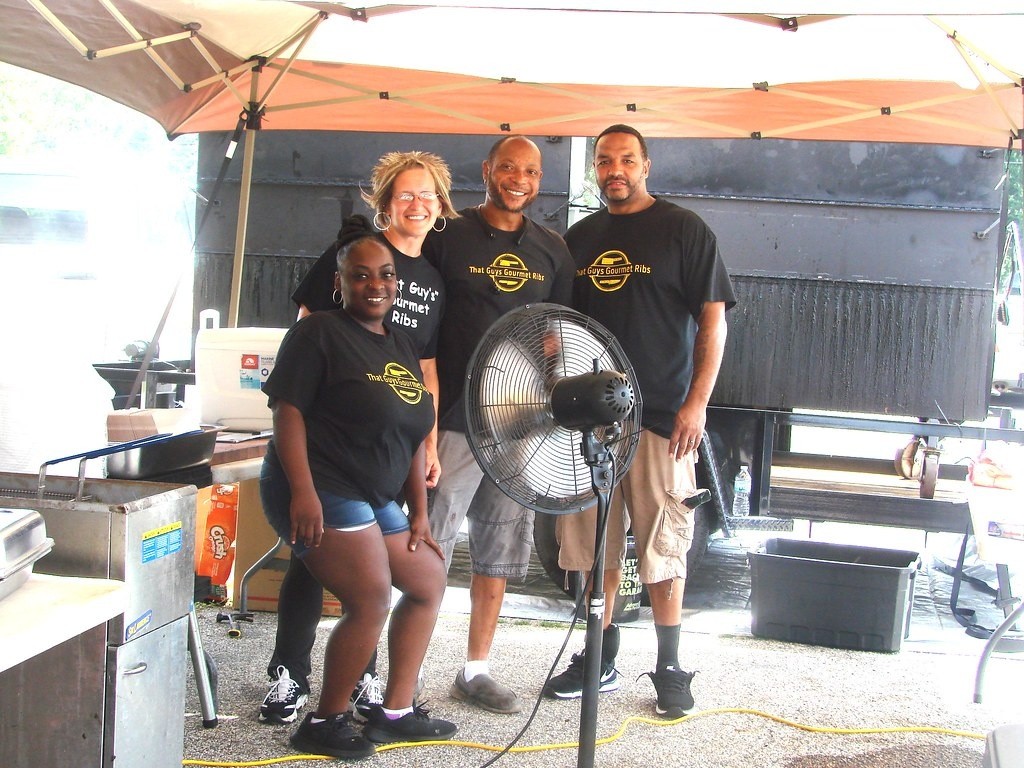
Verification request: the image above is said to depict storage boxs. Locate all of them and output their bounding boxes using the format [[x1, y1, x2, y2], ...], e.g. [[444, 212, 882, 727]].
[[233, 479, 344, 618], [747, 539, 921, 652]]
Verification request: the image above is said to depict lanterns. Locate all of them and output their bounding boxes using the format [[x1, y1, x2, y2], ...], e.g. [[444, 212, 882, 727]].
[[0, 0, 1023, 333]]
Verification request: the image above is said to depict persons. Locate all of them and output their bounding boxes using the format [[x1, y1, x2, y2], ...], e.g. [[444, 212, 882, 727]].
[[538, 124, 740, 719], [255, 151, 460, 724], [332, 136, 576, 715], [259, 239, 457, 762]]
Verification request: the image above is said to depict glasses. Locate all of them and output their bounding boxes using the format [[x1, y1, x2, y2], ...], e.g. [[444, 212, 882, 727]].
[[391, 192, 440, 201]]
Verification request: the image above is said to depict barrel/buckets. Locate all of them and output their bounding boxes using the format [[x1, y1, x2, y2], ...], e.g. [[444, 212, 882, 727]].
[[572, 534, 643, 624]]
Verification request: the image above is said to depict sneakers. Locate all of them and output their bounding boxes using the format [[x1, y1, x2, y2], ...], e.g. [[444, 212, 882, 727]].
[[360, 702, 456, 743], [350, 678, 384, 723], [649, 664, 697, 716], [288, 713, 377, 759], [258, 680, 308, 724], [451, 668, 520, 714], [544, 650, 619, 699]]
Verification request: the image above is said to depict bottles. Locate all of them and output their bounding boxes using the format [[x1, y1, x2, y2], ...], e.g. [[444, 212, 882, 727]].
[[732, 465, 752, 517]]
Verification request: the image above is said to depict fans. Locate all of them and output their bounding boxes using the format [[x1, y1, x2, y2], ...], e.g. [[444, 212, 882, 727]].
[[464, 303, 643, 768]]
